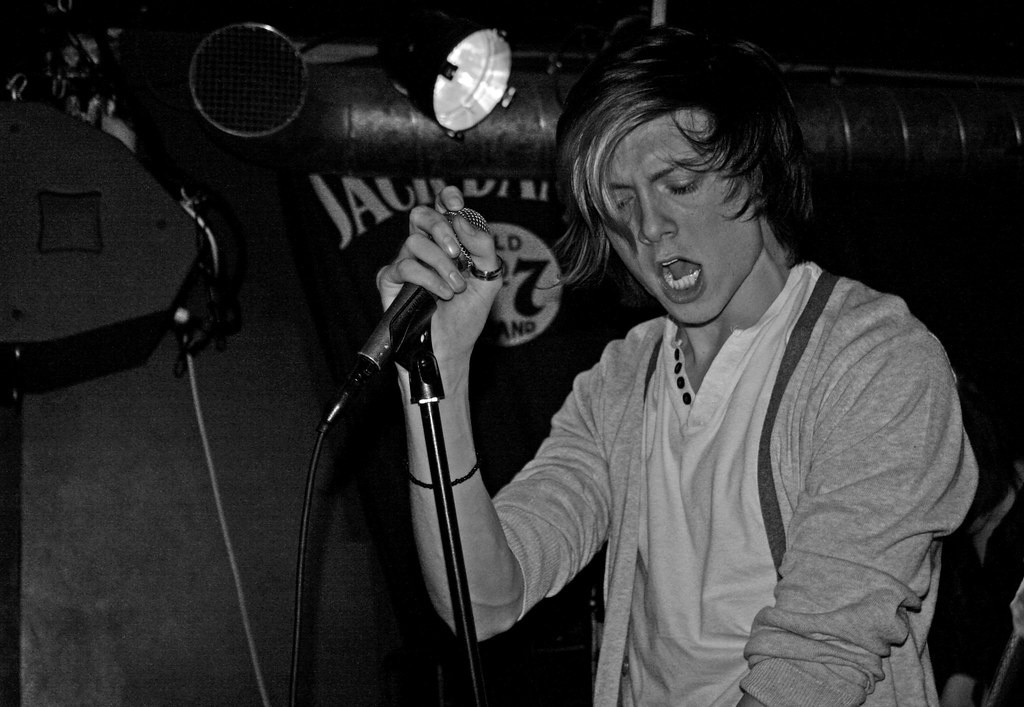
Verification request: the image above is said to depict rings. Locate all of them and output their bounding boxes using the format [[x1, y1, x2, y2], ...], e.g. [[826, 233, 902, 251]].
[[470, 255, 507, 281]]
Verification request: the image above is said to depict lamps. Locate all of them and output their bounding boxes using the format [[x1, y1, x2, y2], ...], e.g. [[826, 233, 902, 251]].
[[372, 0, 517, 141]]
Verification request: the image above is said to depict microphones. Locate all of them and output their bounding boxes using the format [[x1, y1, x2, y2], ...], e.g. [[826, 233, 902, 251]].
[[320, 207, 491, 432]]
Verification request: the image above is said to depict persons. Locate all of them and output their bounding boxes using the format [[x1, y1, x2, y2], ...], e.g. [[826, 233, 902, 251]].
[[375, 25, 980, 707]]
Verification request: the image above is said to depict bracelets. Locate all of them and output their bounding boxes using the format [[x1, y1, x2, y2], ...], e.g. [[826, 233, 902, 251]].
[[403, 449, 482, 489]]
